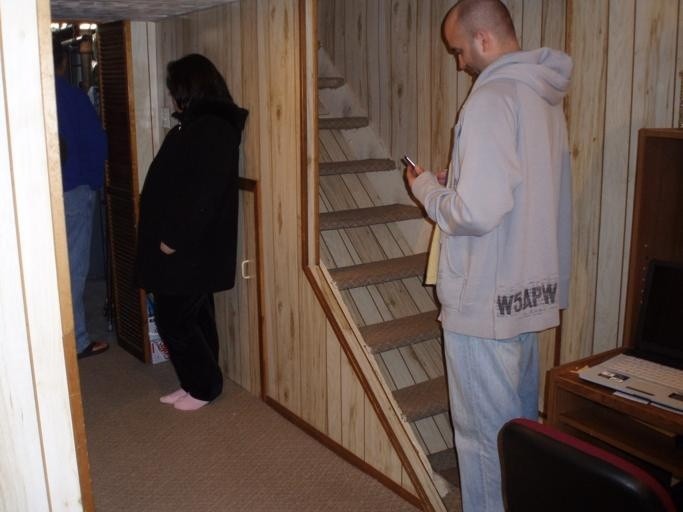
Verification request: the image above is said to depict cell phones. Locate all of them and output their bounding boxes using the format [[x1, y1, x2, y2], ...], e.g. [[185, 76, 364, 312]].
[[403, 153, 417, 170]]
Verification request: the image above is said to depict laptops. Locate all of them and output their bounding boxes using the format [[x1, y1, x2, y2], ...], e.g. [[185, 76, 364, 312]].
[[577, 258, 683, 413]]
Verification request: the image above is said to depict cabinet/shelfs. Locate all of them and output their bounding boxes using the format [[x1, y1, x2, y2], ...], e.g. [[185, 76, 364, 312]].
[[542, 128, 683, 481]]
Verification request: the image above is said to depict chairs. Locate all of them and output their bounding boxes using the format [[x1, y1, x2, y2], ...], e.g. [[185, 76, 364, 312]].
[[496, 416, 677, 512]]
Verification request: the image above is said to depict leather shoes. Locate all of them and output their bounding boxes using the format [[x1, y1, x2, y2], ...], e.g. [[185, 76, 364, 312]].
[[174, 392, 210, 411], [160, 388, 187, 404]]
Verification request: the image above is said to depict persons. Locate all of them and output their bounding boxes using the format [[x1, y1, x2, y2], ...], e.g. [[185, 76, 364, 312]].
[[132, 54, 249, 412], [400, 2, 574, 512], [51, 37, 110, 360]]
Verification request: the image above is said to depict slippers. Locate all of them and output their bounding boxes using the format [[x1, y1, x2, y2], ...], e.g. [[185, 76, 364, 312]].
[[77, 342, 108, 359]]
